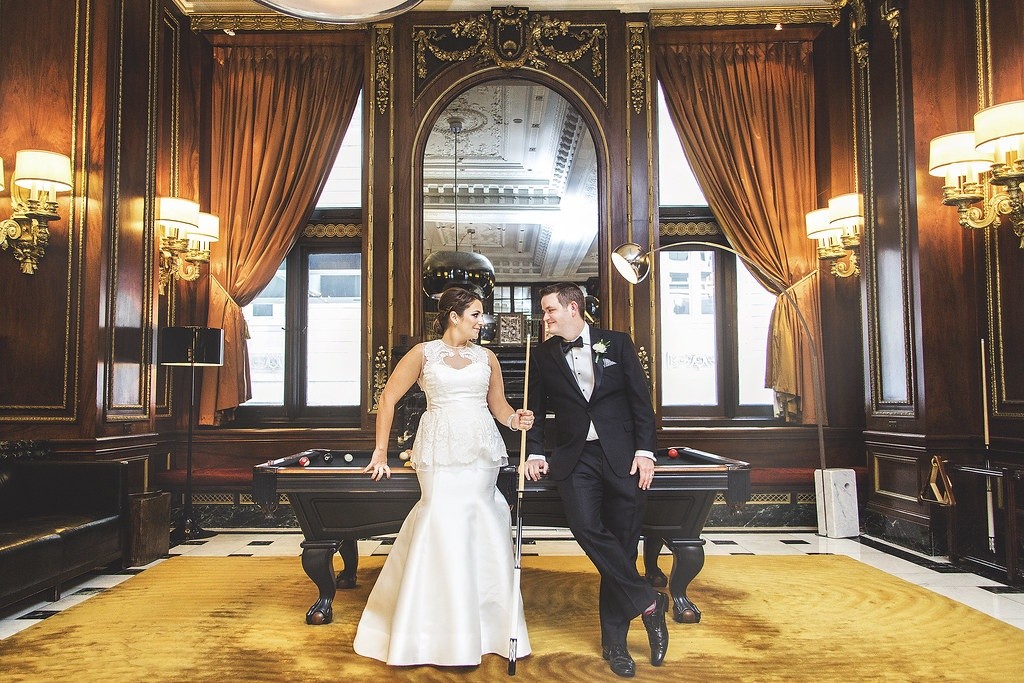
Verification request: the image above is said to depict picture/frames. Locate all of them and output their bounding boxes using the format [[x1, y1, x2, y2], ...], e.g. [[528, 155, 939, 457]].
[[542, 320, 554, 344], [425, 312, 443, 342], [497, 312, 524, 346], [480, 323, 497, 346], [524, 318, 540, 343]]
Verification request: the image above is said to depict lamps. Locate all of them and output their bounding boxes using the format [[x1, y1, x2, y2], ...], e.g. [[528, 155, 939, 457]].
[[421, 115, 497, 300], [255, 0, 425, 26], [159, 195, 223, 296], [160, 325, 226, 543], [805, 192, 865, 279], [928, 98, 1024, 251], [0, 148, 76, 276], [611, 240, 860, 540]]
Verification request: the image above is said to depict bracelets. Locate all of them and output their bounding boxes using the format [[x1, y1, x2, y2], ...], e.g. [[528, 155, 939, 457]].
[[507, 414, 518, 431]]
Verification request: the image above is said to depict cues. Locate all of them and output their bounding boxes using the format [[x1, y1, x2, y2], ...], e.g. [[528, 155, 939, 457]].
[[506, 332, 531, 676], [979, 338, 998, 557]]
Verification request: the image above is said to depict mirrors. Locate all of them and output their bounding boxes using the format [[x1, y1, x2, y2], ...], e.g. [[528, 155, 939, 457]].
[[410, 63, 613, 346]]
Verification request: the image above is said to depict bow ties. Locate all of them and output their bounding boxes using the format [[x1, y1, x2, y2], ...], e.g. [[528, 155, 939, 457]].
[[560, 335, 585, 354]]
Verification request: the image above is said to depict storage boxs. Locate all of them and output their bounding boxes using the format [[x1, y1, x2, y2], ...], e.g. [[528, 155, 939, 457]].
[[127, 491, 172, 566]]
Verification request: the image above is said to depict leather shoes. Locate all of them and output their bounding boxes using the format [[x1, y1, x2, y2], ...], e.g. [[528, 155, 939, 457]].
[[643, 588, 670, 667], [602, 641, 638, 676]]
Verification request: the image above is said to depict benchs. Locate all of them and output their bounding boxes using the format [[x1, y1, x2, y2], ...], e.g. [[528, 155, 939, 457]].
[[0, 458, 123, 615]]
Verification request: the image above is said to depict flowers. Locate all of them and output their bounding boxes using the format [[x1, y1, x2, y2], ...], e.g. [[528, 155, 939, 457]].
[[592, 338, 611, 363]]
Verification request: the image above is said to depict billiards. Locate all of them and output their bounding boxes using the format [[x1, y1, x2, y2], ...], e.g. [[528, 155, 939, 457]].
[[344, 453, 354, 463], [398, 449, 412, 460], [323, 453, 333, 463], [668, 448, 678, 458], [404, 461, 411, 467], [299, 457, 311, 467]]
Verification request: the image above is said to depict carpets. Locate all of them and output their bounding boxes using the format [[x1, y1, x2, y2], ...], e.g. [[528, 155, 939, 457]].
[[0, 553, 1024, 683]]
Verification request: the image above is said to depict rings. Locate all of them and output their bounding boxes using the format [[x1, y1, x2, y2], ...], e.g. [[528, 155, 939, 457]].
[[650, 474, 653, 476]]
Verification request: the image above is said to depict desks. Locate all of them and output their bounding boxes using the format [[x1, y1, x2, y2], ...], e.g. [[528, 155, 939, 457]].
[[252, 446, 752, 628]]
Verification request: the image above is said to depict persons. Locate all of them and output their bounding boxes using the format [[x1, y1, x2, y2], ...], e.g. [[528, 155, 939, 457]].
[[352, 288, 535, 666], [518, 281, 669, 678]]
